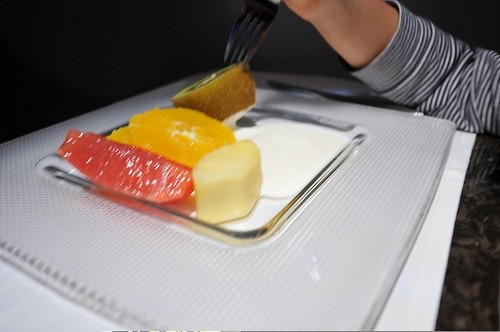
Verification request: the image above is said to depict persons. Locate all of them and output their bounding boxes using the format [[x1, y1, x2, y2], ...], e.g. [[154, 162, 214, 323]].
[[283, 0, 500, 136]]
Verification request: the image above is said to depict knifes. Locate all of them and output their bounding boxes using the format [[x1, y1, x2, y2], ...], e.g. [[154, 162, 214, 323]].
[[268, 80, 415, 110]]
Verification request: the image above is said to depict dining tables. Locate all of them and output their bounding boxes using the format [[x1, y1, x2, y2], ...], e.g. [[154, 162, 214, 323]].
[[0, 69, 477, 332]]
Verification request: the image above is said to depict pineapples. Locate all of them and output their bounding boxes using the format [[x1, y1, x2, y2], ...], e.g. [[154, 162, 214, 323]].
[[104, 108, 237, 170]]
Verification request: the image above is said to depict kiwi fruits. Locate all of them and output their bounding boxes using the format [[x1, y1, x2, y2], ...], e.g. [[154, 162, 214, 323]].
[[170, 59, 256, 122]]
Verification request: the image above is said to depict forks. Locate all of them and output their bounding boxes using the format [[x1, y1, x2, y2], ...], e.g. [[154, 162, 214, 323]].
[[224, 0, 283, 66]]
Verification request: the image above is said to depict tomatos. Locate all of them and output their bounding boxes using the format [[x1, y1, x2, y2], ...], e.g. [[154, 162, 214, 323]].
[[54, 127, 194, 205]]
